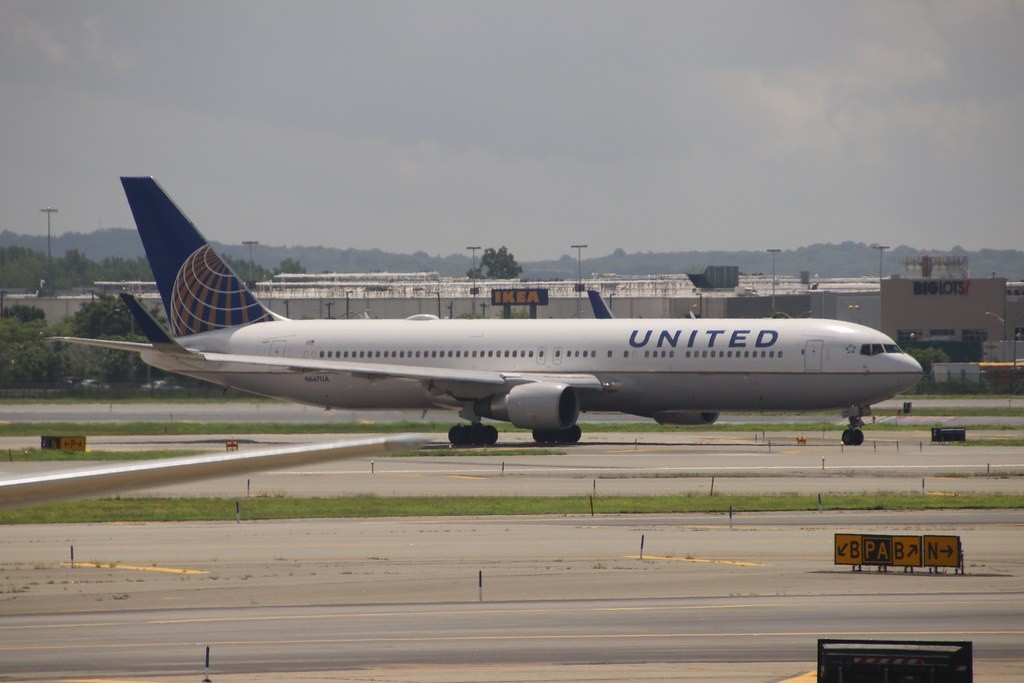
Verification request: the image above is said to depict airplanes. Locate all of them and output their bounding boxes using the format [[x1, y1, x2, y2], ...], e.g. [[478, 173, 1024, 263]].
[[40, 176, 924, 448]]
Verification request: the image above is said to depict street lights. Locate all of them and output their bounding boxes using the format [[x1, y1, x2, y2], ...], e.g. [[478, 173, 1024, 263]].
[[572, 244, 589, 318], [419, 288, 425, 314], [283, 299, 289, 319], [696, 293, 702, 318], [766, 249, 782, 318], [325, 303, 331, 319], [242, 240, 260, 282], [346, 290, 354, 319], [985, 288, 1009, 363], [467, 245, 482, 318], [433, 291, 442, 320], [40, 207, 58, 296], [873, 246, 890, 332], [610, 293, 615, 310]]
